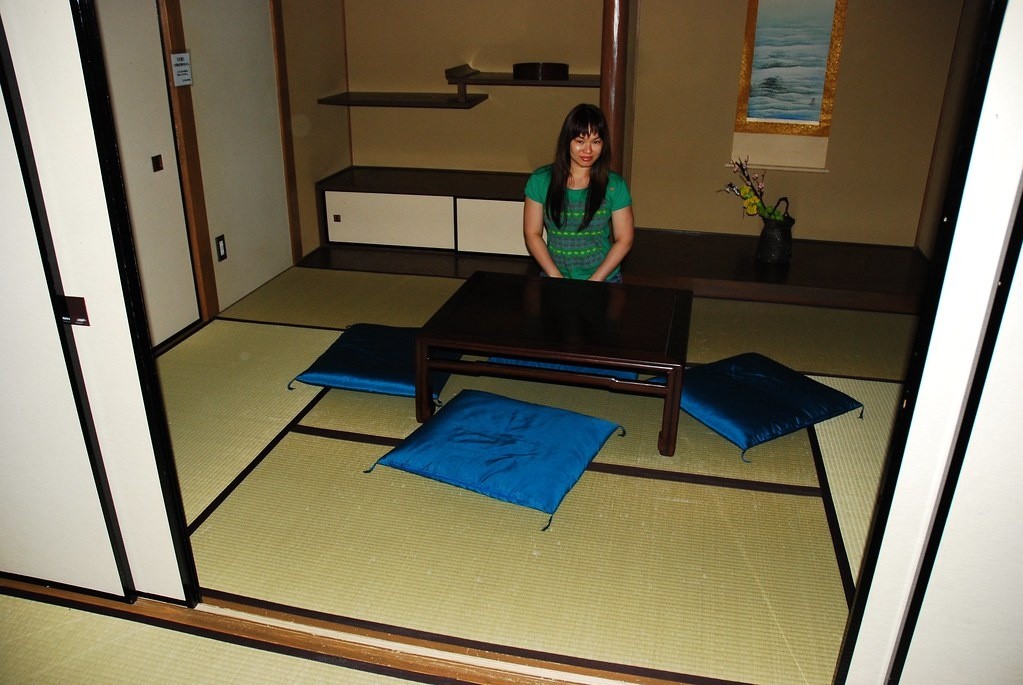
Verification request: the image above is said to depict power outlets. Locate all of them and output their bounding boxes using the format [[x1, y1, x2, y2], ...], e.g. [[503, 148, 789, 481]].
[[215, 234, 227, 262]]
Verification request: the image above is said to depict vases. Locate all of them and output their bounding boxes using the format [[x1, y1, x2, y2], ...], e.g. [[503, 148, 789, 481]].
[[754, 215, 792, 285]]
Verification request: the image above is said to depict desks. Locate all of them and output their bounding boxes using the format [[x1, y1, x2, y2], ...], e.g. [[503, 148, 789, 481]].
[[416, 268, 694, 457]]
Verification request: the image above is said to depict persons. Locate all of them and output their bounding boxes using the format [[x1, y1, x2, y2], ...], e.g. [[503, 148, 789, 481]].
[[523, 103, 635, 283]]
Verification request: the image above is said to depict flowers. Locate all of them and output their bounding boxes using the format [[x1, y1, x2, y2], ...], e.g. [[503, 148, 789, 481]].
[[718, 155, 786, 221]]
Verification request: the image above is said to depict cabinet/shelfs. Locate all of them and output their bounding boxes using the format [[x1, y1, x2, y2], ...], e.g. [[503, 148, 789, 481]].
[[315, 164, 549, 262], [317, 71, 602, 110]]
